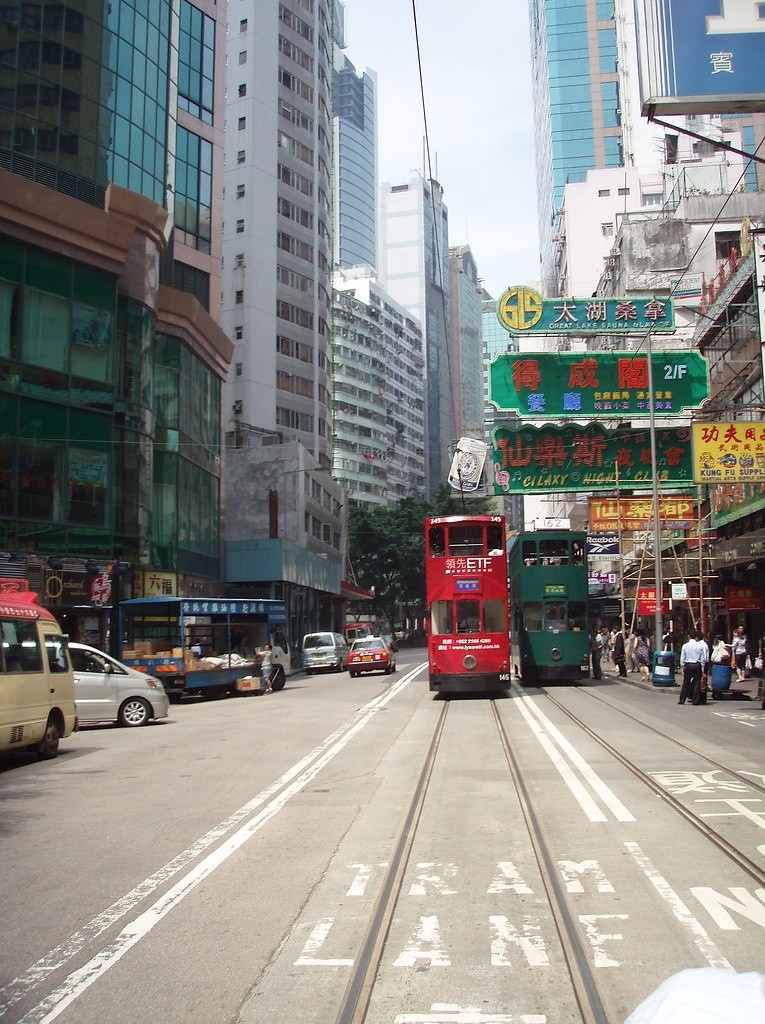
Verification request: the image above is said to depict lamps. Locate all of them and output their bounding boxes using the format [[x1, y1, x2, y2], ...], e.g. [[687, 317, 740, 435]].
[[46, 556, 62, 570], [85, 562, 98, 575], [7, 554, 23, 563], [116, 565, 129, 575]]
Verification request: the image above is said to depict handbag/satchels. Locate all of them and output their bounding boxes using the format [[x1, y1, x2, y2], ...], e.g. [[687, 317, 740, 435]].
[[710, 641, 724, 663], [755, 657, 763, 669], [700, 673, 707, 692], [745, 655, 753, 669]]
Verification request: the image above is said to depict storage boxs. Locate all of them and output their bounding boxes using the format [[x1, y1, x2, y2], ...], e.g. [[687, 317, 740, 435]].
[[121, 641, 260, 692]]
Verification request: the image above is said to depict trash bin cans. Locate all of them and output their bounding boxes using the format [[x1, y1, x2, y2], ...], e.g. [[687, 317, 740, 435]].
[[651, 650, 677, 687]]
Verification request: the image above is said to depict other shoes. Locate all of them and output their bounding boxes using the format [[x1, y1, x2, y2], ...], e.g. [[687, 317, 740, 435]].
[[623, 675, 627, 677], [618, 674, 622, 676], [678, 702, 684, 704], [647, 673, 650, 681], [730, 653, 735, 669], [736, 676, 744, 683], [688, 699, 692, 702], [637, 670, 640, 672], [265, 690, 273, 695], [592, 676, 601, 680], [640, 676, 645, 681]]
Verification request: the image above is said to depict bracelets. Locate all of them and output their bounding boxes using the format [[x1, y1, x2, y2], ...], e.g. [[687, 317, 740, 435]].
[[759, 653, 761, 656]]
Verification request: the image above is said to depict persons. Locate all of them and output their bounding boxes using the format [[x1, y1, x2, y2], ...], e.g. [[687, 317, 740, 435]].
[[610, 626, 627, 677], [625, 629, 639, 673], [732, 625, 751, 678], [725, 629, 746, 682], [590, 630, 603, 680], [649, 628, 656, 655], [678, 632, 705, 705], [257, 644, 273, 693], [602, 628, 611, 662], [190, 639, 205, 656], [712, 635, 730, 665], [759, 631, 765, 678], [634, 629, 650, 681], [688, 632, 709, 705]]
